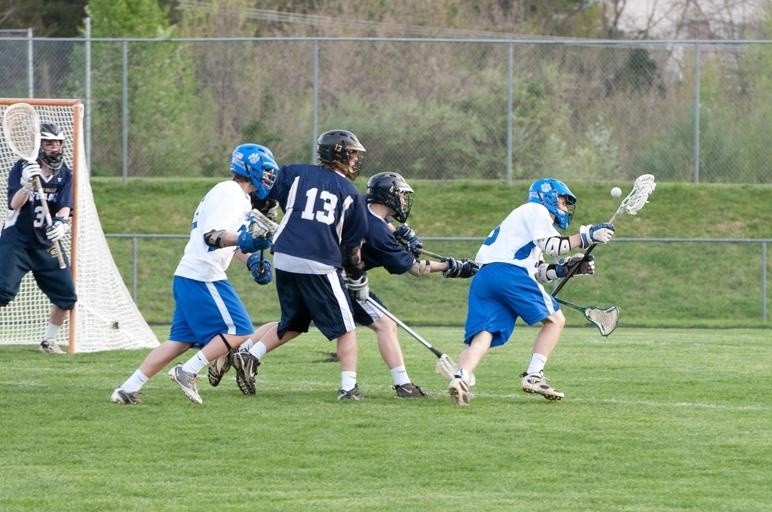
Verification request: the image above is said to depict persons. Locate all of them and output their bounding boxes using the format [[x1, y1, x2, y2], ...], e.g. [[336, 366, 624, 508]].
[[0, 122, 78, 357], [207, 170, 477, 398], [447, 177, 613, 407], [233, 127, 366, 400], [110, 142, 279, 406]]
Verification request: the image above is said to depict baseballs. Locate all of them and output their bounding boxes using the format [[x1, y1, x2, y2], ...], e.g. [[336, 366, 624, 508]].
[[611, 187, 622, 198]]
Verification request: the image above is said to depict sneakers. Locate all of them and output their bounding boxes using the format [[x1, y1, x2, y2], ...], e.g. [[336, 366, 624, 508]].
[[336, 382, 376, 401], [392, 382, 434, 400], [38, 339, 68, 355], [448, 375, 473, 407], [109, 386, 145, 405], [167, 362, 203, 405], [522, 370, 565, 401], [230, 351, 261, 395], [208, 345, 240, 386]]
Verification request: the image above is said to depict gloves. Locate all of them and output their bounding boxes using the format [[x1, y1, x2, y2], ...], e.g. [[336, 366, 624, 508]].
[[233, 224, 273, 256], [579, 223, 616, 251], [247, 252, 272, 285], [440, 257, 479, 278], [392, 223, 424, 258], [20, 161, 42, 188], [346, 275, 369, 301], [556, 253, 595, 278], [45, 217, 70, 242]]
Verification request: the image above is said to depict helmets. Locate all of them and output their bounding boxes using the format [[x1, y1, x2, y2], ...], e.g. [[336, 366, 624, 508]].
[[527, 178, 577, 230], [229, 143, 280, 200], [366, 172, 415, 223], [38, 124, 66, 170], [316, 130, 367, 181]]
